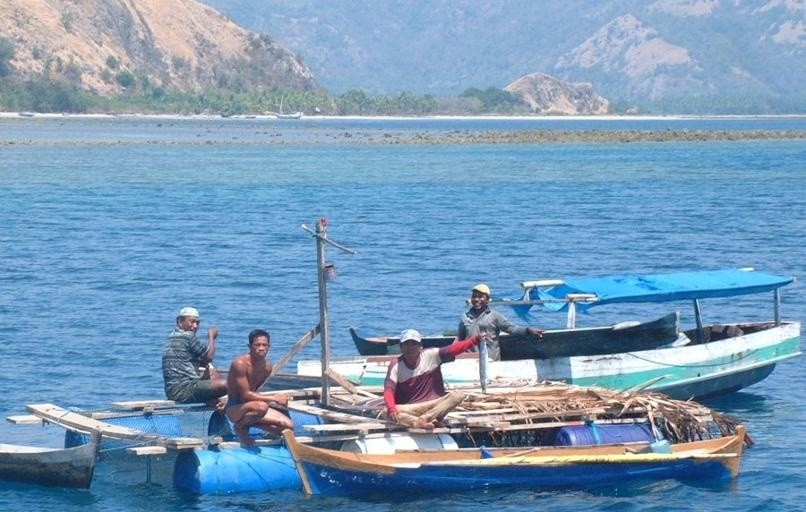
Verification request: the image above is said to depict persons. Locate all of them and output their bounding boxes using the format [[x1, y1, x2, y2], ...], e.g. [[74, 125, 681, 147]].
[[381, 329, 493, 432], [224, 330, 294, 446], [452, 284, 544, 361], [162, 307, 228, 408]]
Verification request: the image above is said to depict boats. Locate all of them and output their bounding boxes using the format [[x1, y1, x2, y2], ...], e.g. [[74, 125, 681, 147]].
[[296, 263, 801, 402], [278, 421, 748, 500]]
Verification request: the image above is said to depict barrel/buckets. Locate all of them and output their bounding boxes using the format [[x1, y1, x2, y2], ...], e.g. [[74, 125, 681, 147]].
[[65, 414, 183, 454], [208, 402, 333, 443], [339, 435, 460, 457], [173, 448, 320, 496], [557, 426, 660, 446]]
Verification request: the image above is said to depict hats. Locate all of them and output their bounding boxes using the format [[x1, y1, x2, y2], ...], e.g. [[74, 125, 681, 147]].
[[472, 284, 490, 297], [179, 307, 199, 317], [400, 330, 422, 343]]
